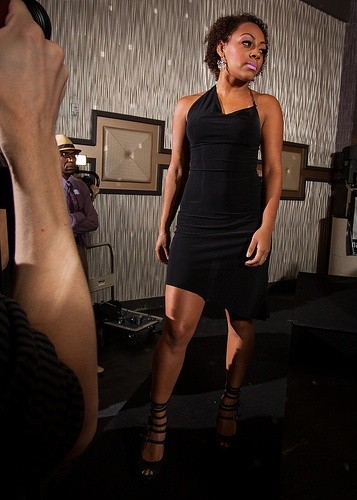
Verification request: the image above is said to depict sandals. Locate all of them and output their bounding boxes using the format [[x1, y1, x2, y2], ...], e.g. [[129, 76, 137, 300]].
[[215, 383, 242, 452], [135, 401, 170, 485]]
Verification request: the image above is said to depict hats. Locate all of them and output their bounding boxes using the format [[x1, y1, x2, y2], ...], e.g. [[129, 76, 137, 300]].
[[54, 134, 81, 155]]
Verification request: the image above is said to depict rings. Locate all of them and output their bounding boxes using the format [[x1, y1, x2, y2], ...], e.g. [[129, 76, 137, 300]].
[[265, 257, 267, 260]]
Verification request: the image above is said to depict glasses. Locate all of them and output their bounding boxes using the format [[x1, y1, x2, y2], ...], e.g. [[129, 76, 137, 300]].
[[62, 151, 76, 159]]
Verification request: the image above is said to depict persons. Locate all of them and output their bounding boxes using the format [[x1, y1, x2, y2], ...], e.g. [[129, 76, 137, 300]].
[[132, 13, 286, 478], [0, 0, 99, 500], [51, 135, 104, 374]]
